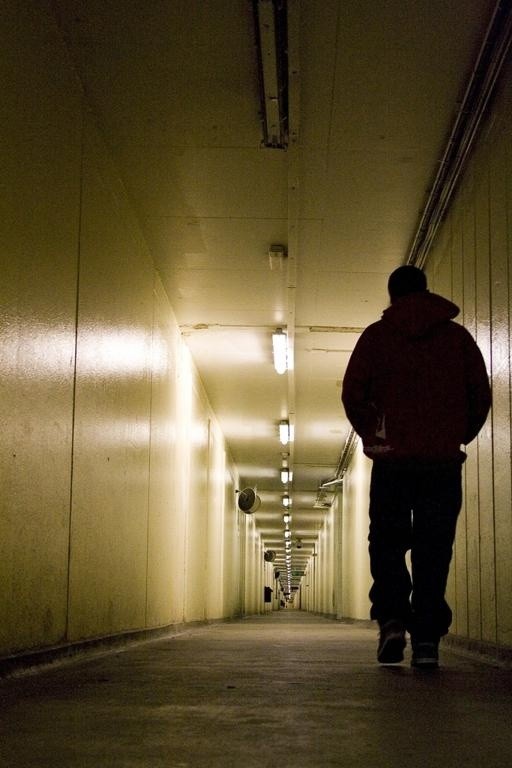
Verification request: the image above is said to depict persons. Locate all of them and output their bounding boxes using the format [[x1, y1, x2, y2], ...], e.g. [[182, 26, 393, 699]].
[[340, 265, 494, 671]]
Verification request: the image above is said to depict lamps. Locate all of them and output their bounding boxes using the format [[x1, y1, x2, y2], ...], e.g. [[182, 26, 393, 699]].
[[254, 0, 292, 602]]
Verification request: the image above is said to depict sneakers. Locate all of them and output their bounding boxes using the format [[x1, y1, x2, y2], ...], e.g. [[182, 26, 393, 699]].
[[411, 642, 439, 663], [377, 620, 406, 663]]
